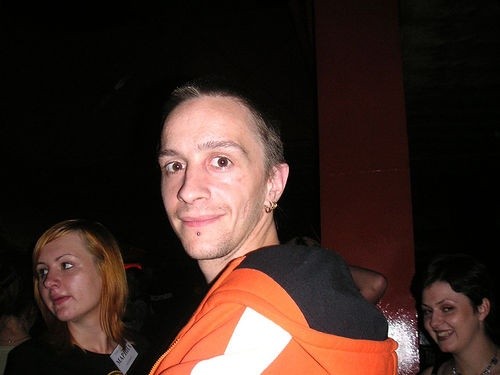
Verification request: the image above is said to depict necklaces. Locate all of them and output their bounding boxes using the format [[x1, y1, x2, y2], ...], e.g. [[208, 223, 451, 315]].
[[451, 355, 498, 375]]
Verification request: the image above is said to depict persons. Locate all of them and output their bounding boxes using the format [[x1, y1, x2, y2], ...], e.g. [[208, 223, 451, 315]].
[[148, 85, 400, 375], [0, 220, 177, 375], [409, 255, 500, 375], [287, 236, 387, 305]]
